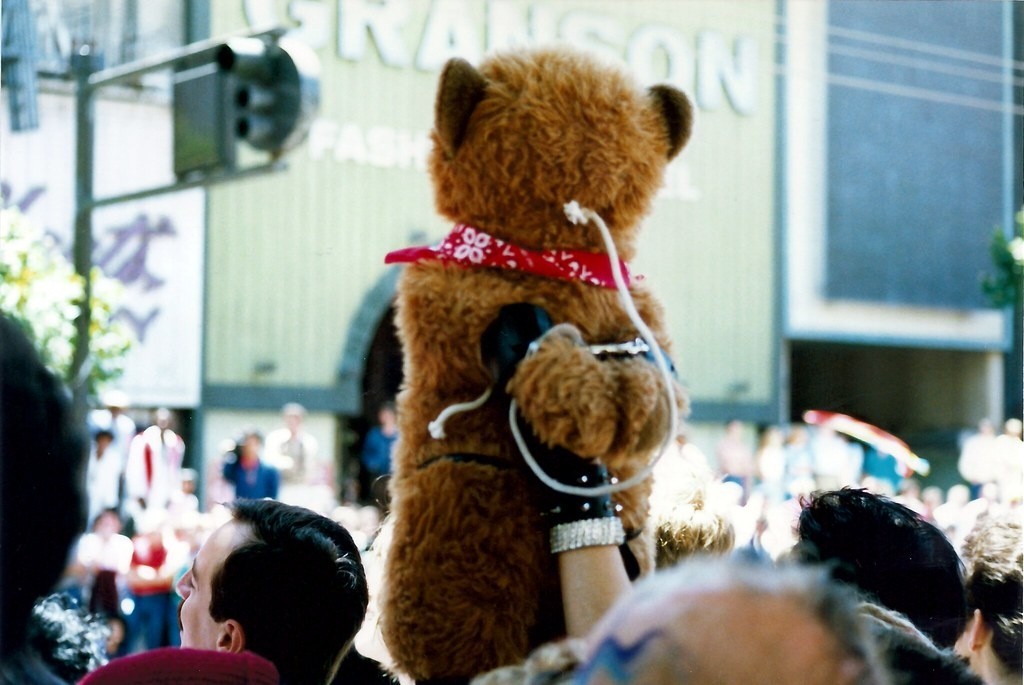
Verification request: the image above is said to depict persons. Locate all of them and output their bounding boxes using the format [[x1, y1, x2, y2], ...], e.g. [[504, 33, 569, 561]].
[[172, 497, 397, 684], [566, 553, 883, 684], [541, 450, 630, 642], [950, 501, 1023, 685], [791, 485, 966, 652], [649, 498, 737, 571], [62, 399, 395, 656], [1, 310, 84, 684], [661, 413, 1023, 560]]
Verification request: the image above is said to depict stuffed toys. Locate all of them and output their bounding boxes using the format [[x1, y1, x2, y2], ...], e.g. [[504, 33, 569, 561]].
[[382, 46, 691, 684]]
